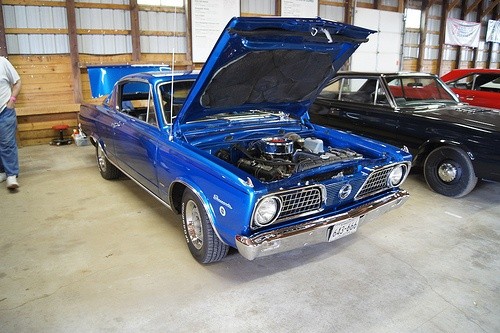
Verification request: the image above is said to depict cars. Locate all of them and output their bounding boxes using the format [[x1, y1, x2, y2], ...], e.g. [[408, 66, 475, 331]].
[[388, 68, 500, 110], [308, 71, 500, 199], [77, 16, 413, 264]]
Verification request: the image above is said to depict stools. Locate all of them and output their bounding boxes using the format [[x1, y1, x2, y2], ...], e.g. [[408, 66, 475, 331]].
[[50, 125, 73, 147]]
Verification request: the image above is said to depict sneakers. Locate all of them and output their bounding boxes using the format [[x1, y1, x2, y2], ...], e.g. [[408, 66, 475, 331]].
[[6, 175, 19, 189], [0, 172, 6, 183]]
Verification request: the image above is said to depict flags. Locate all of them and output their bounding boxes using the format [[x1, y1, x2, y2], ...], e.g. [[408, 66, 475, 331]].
[[486, 20, 500, 42], [445, 17, 481, 48]]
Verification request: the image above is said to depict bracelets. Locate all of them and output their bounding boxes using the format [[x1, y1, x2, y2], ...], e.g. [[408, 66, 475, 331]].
[[10, 97, 16, 102]]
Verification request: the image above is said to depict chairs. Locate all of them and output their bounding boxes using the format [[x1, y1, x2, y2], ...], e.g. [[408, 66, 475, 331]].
[[126, 106, 155, 119], [172, 103, 183, 117], [138, 110, 172, 124]]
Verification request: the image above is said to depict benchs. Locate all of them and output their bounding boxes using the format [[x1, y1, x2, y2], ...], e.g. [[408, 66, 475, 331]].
[[337, 91, 373, 103], [453, 83, 470, 89]]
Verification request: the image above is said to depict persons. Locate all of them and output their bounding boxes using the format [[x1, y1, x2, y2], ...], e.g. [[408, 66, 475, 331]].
[[143, 137, 170, 170], [0, 57, 21, 189]]
